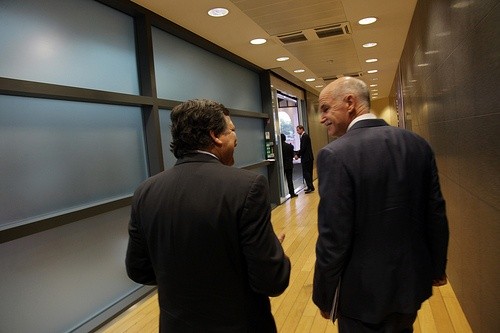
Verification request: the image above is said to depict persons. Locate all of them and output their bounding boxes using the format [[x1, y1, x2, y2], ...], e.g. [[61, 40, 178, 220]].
[[125, 99, 292, 333], [312, 76, 449, 333], [280, 134, 298, 197], [294, 126, 315, 194]]
